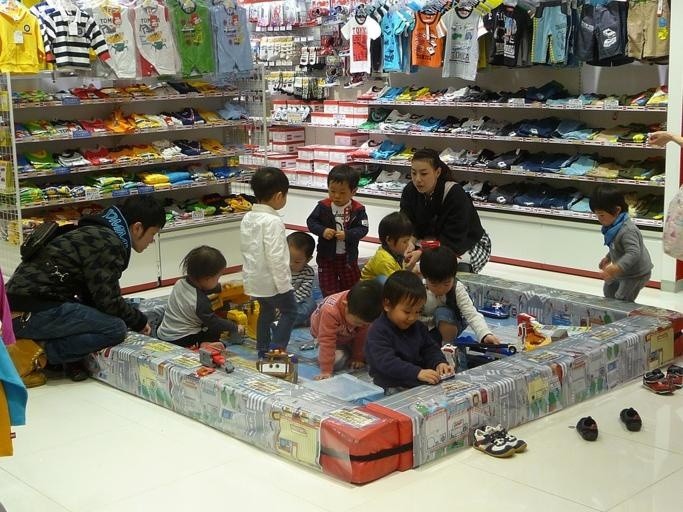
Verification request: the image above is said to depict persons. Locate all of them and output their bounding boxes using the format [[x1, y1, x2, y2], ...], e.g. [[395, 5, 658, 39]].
[[412, 247, 505, 351], [587, 182, 654, 302], [646, 130, 683, 151], [285, 231, 316, 329], [358, 209, 414, 281], [305, 163, 369, 298], [156, 245, 246, 347], [309, 278, 383, 381], [361, 269, 454, 396], [239, 166, 299, 363], [4, 194, 167, 383], [396, 146, 491, 274]]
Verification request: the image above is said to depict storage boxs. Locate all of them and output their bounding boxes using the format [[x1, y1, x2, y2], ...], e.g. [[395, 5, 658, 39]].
[[242, 96, 370, 190]]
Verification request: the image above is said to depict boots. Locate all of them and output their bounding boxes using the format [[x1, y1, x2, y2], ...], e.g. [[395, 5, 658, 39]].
[[5, 337, 48, 388]]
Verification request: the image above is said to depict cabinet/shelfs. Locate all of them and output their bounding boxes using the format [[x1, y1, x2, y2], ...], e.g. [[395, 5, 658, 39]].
[[0, 62, 269, 298], [351, 100, 667, 240]]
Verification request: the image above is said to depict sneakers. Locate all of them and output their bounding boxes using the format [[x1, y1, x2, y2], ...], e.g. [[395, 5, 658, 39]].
[[641, 367, 676, 396], [63, 362, 89, 382], [481, 423, 528, 454], [619, 407, 641, 432], [471, 428, 515, 458], [665, 363, 683, 388], [575, 415, 599, 442]]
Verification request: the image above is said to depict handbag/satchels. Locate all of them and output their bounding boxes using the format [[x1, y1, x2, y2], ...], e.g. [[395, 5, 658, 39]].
[[18, 219, 61, 264]]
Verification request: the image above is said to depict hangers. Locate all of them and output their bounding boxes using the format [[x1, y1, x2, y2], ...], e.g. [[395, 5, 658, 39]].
[[351, 1, 516, 20]]
